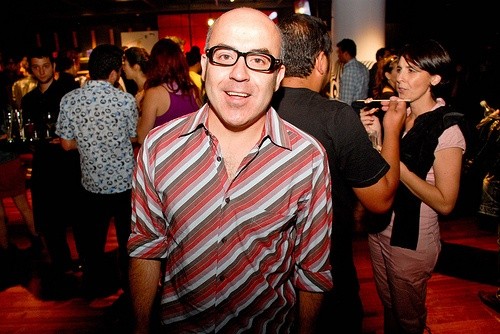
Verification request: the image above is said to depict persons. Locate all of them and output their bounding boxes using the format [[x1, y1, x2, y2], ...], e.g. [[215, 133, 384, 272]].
[[22, 50, 80, 269], [377, 56, 400, 142], [137, 39, 203, 144], [127, 6, 333, 334], [186, 51, 205, 96], [55, 46, 139, 285], [336, 39, 370, 107], [369, 49, 392, 98], [0, 49, 85, 111], [272, 14, 406, 334], [0, 88, 44, 261], [359, 36, 466, 334], [123, 47, 149, 108]]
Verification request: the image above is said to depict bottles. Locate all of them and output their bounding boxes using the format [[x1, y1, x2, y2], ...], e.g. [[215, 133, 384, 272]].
[[25, 118, 35, 140], [7, 106, 20, 141], [46, 111, 55, 138]]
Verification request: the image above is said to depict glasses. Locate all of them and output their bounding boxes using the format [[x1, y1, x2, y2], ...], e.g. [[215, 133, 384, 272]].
[[206, 46, 283, 71]]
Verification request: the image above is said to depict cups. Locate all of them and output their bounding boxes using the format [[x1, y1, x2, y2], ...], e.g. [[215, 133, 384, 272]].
[[368, 130, 379, 147]]
